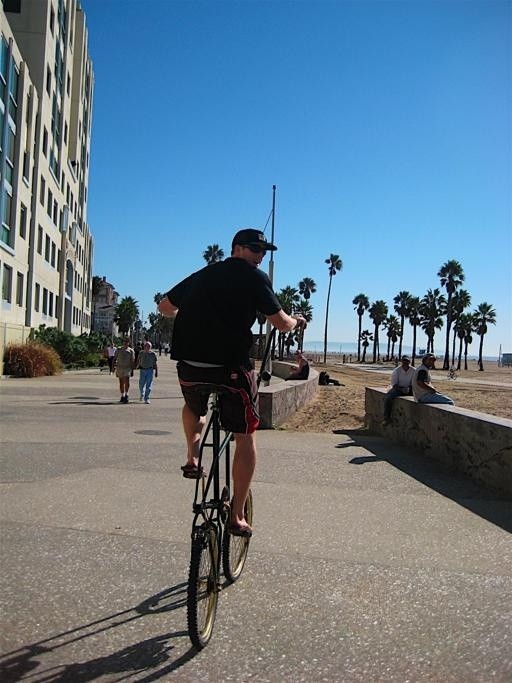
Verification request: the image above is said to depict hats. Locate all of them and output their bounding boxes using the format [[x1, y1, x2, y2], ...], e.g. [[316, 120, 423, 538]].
[[233, 229, 277, 249], [422, 353, 436, 360], [402, 355, 410, 361]]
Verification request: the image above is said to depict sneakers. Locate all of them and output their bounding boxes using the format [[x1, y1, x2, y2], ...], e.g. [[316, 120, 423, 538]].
[[124, 395, 129, 404], [382, 418, 392, 426], [120, 396, 125, 403], [146, 399, 150, 404], [140, 395, 144, 401]]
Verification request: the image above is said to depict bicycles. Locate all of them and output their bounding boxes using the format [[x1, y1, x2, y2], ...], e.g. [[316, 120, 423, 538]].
[[187, 312, 307, 652], [445, 369, 457, 381]]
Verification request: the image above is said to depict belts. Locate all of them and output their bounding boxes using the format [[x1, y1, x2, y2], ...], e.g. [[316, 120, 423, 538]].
[[141, 367, 153, 370]]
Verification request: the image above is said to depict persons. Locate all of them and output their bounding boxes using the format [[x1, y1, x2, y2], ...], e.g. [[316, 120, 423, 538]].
[[94, 331, 177, 406], [380, 354, 416, 428], [317, 369, 345, 387], [156, 227, 300, 540], [410, 352, 457, 407], [335, 347, 411, 364], [284, 350, 309, 380]]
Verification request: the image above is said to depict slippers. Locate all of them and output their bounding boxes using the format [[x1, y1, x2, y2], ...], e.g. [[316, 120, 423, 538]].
[[229, 522, 254, 536], [181, 464, 208, 477]]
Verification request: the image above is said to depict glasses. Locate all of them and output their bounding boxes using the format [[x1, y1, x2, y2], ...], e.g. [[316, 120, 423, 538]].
[[242, 246, 267, 255]]
